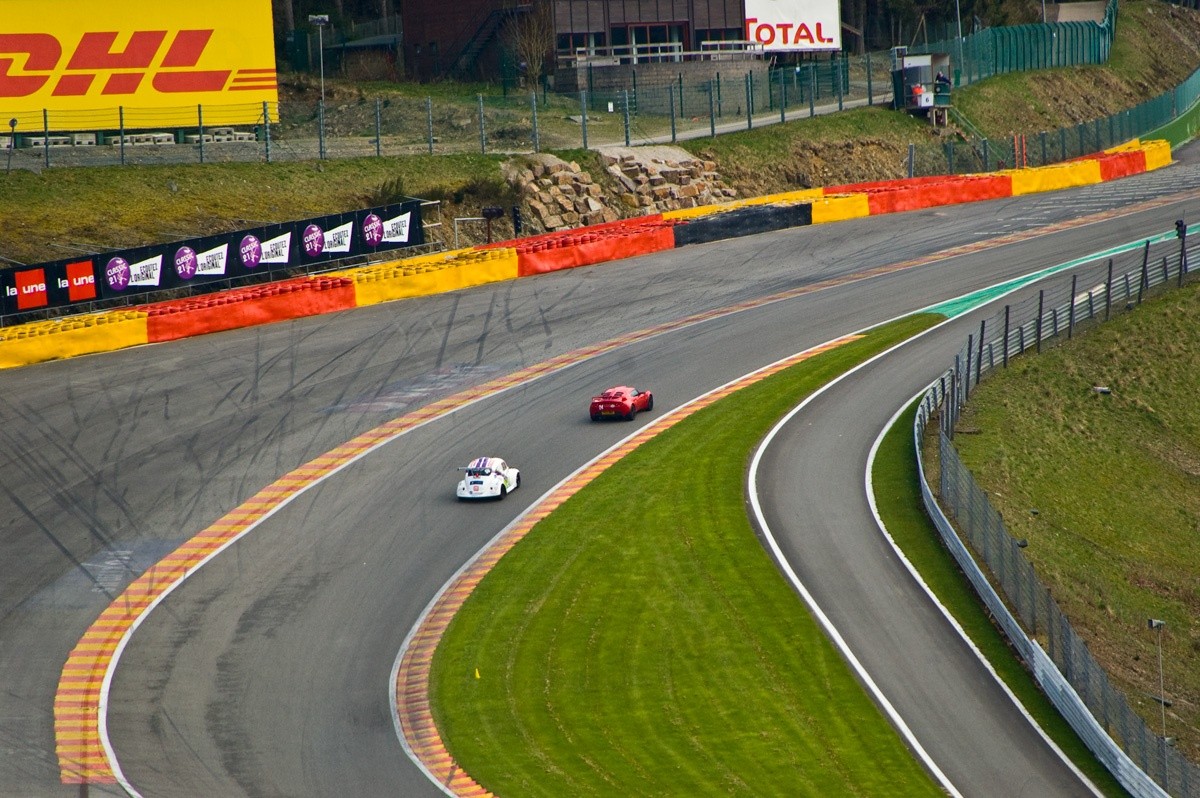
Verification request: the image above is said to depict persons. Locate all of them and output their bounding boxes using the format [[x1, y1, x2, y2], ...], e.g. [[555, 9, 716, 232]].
[[911, 83, 924, 104], [935, 71, 952, 87]]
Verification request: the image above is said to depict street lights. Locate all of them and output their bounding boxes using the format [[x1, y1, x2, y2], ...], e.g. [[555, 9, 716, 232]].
[[308, 14, 330, 101]]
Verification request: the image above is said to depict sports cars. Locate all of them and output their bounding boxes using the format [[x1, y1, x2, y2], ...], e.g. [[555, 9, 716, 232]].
[[456, 457, 520, 501], [588, 386, 653, 421]]
